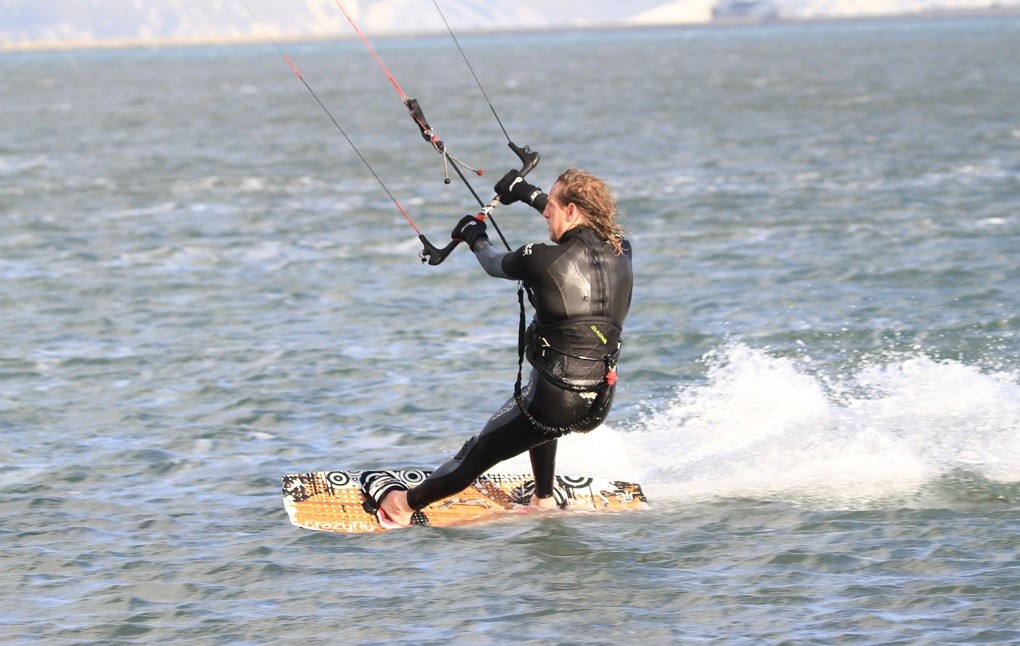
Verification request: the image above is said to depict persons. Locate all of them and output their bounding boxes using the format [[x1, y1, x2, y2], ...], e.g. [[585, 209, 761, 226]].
[[362, 167, 634, 530]]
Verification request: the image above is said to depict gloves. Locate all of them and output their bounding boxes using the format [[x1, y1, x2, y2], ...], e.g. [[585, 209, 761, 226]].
[[495, 169, 541, 205], [452, 215, 489, 251]]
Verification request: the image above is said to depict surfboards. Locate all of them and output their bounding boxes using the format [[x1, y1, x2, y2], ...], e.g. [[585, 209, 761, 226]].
[[281, 469, 650, 534]]
